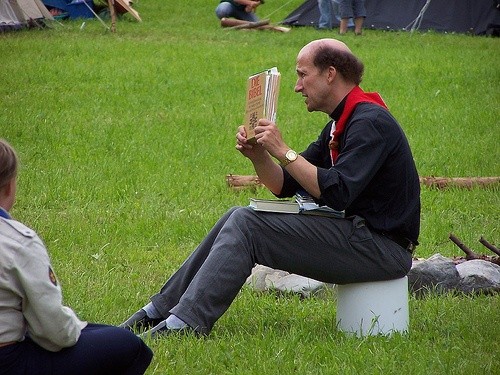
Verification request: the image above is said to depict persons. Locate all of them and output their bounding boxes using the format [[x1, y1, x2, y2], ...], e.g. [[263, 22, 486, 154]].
[[117, 37, 421, 342], [216, 0, 367, 35], [0, 139, 154, 375]]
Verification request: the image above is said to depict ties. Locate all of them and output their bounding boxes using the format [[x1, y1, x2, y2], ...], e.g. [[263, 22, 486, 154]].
[[329, 86, 388, 166]]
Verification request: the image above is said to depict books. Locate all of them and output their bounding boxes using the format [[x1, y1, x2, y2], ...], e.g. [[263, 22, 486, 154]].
[[249, 191, 347, 218], [244, 66, 280, 144]]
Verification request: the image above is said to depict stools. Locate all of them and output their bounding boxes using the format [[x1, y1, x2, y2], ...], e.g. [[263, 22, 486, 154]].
[[337, 275, 409, 339]]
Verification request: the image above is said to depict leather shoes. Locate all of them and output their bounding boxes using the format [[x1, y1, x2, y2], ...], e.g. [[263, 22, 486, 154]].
[[118, 308, 166, 330], [135, 320, 195, 342]]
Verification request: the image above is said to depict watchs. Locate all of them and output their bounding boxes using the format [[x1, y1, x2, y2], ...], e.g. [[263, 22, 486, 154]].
[[280, 149, 299, 167]]
